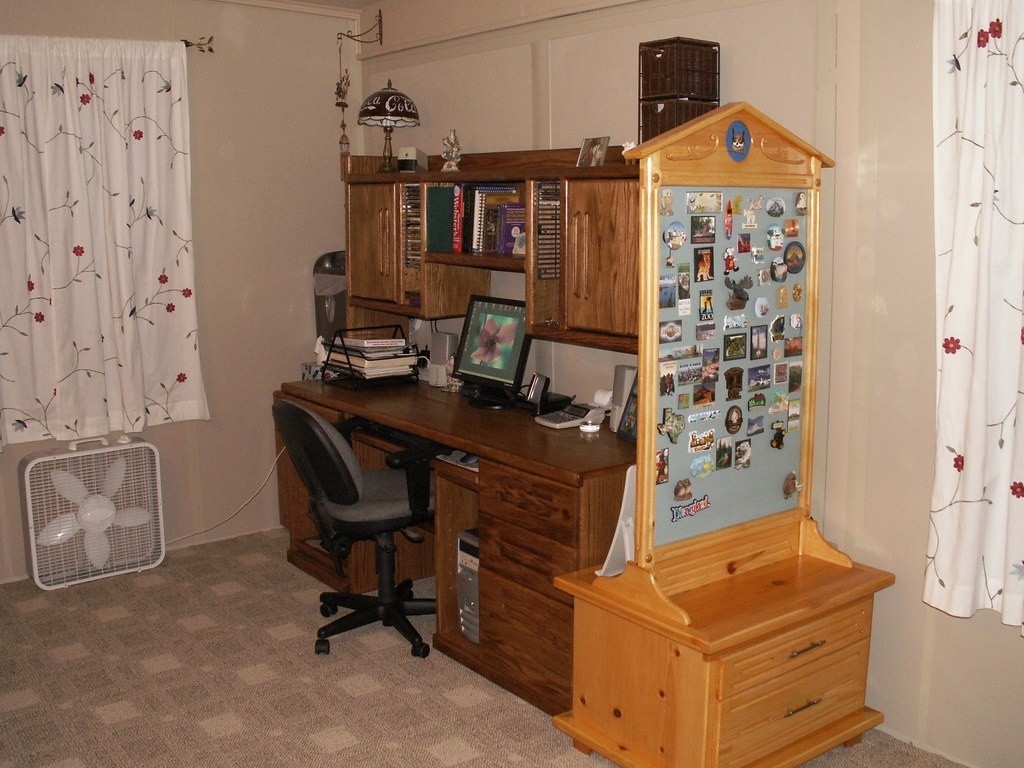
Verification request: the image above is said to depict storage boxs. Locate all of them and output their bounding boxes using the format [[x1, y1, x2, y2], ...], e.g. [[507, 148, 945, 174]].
[[638, 38, 720, 145]]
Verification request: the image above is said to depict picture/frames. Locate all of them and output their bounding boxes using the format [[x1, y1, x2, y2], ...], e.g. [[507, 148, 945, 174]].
[[575, 137, 611, 167]]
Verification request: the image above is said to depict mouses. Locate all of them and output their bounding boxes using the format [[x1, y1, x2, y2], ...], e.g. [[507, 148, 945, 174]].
[[459, 453, 481, 463]]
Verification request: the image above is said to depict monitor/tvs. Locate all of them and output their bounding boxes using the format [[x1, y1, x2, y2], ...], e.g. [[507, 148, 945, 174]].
[[452, 294, 532, 410]]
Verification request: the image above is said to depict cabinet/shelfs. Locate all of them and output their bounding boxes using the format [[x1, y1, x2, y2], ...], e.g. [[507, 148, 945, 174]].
[[339, 145, 637, 380], [552, 558, 895, 768]]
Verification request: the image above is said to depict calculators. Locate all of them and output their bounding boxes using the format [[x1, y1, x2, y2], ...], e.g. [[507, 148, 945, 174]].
[[534, 403, 605, 430]]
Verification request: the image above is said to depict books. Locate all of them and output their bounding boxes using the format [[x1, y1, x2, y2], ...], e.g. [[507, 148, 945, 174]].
[[425, 184, 527, 254], [436, 450, 480, 472], [321, 335, 418, 380]]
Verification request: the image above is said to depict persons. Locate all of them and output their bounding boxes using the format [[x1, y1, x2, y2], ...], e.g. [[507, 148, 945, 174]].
[[443, 129, 461, 168]]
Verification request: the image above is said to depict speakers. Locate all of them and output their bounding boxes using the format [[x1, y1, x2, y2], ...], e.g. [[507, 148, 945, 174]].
[[526, 373, 550, 406], [611, 365, 638, 433], [428, 332, 458, 388]]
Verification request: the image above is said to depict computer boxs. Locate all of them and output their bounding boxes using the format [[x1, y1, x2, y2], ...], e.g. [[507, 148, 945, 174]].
[[456, 528, 481, 644]]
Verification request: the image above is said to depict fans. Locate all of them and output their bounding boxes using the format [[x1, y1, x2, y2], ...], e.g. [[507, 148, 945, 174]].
[[18, 435, 167, 591]]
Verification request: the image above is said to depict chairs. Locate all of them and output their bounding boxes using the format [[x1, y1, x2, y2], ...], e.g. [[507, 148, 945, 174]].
[[271, 399, 437, 658]]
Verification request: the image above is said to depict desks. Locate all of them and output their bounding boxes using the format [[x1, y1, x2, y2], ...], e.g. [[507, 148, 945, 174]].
[[273, 363, 632, 718]]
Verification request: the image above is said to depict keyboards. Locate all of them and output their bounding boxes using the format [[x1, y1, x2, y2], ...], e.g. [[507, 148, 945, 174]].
[[351, 416, 455, 455]]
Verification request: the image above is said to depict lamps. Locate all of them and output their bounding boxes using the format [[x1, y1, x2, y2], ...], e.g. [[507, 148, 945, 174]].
[[357, 78, 419, 173]]
